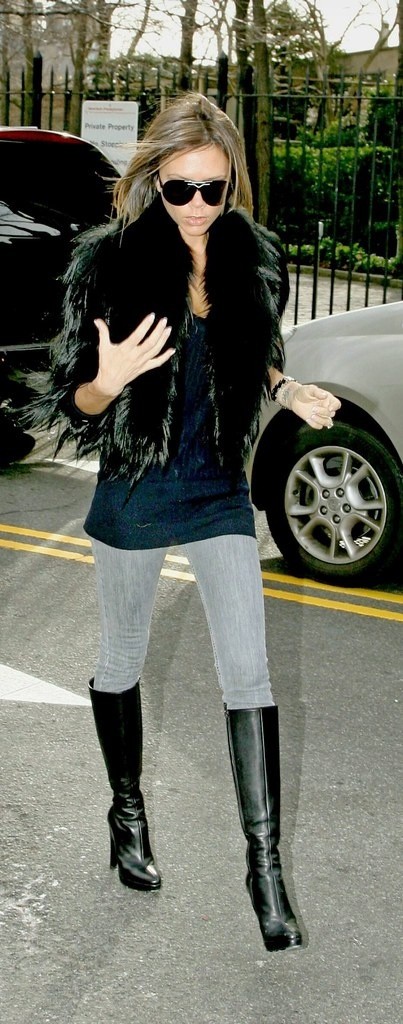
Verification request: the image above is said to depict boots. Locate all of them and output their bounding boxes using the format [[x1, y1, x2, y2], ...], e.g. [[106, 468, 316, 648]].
[[87, 674, 161, 892], [223, 705, 303, 953]]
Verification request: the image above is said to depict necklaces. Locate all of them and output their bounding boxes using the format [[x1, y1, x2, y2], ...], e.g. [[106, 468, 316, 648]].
[[194, 249, 204, 265]]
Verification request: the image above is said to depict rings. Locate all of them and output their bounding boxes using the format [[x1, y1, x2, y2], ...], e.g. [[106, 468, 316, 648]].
[[327, 417, 334, 429]]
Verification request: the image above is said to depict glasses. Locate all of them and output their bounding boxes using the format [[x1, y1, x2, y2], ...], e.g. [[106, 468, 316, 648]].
[[156, 170, 230, 206]]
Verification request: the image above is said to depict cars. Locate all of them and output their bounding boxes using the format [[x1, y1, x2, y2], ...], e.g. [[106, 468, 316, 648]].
[[243, 301, 403, 587]]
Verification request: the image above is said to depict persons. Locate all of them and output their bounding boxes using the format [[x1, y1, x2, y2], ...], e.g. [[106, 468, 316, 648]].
[[48, 90, 344, 954]]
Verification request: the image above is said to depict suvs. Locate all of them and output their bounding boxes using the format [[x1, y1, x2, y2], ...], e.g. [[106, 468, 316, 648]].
[[0, 126, 123, 435]]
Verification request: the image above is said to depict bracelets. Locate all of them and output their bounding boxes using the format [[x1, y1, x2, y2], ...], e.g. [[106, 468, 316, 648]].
[[271, 376, 297, 401]]
